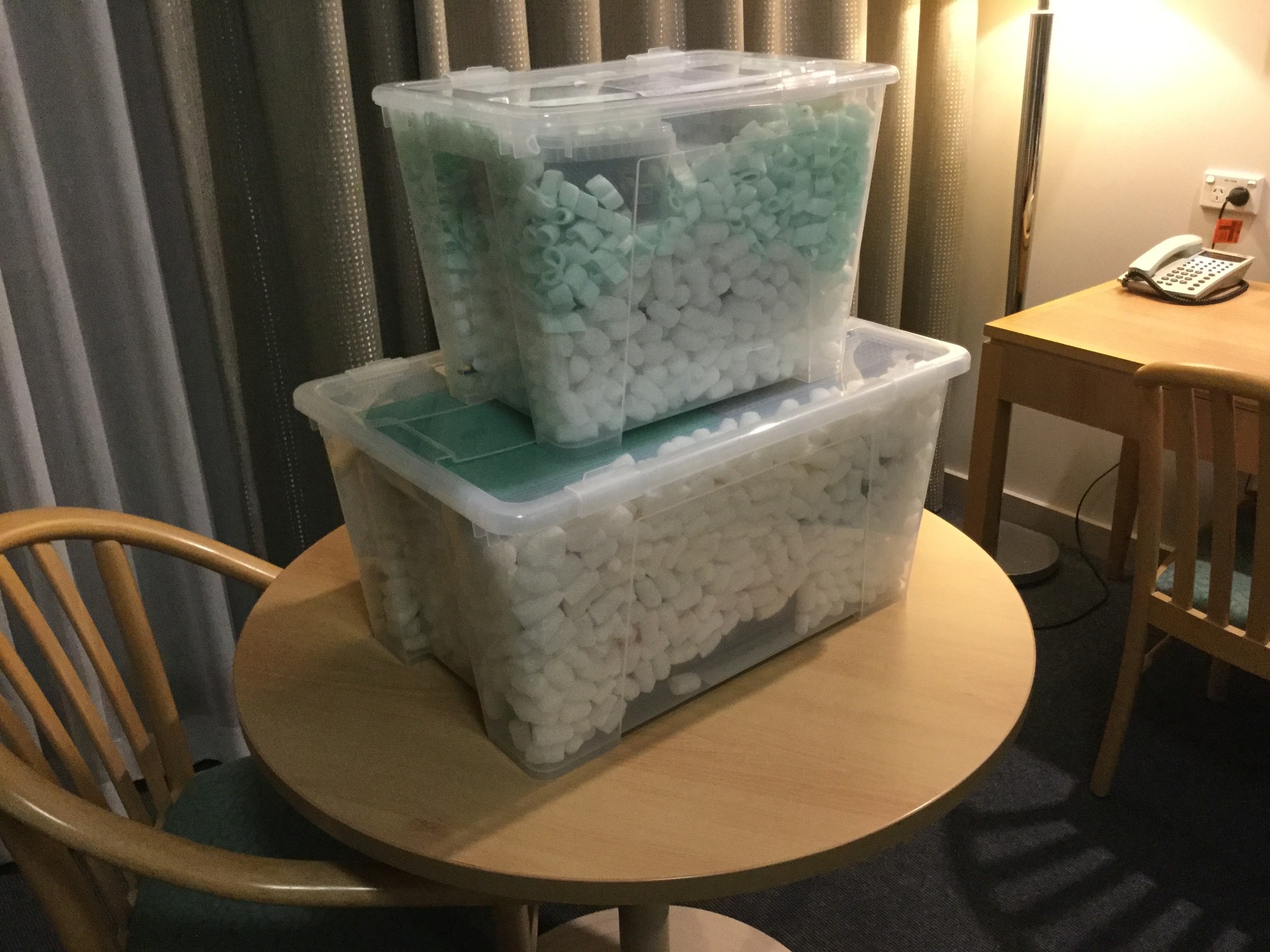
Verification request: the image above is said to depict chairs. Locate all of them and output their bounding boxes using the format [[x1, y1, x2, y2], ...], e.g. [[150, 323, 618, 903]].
[[0, 505, 511, 952], [1091, 363, 1270, 810]]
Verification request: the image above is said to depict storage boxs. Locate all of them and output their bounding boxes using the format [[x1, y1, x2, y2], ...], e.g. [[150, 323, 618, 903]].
[[374, 46, 900, 452], [296, 316, 972, 777]]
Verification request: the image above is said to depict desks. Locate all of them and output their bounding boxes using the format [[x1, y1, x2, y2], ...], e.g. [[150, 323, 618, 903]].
[[960, 278, 1270, 583], [233, 506, 1037, 952]]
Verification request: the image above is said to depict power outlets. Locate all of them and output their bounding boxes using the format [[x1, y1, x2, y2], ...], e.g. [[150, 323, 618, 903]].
[[1198, 169, 1265, 215]]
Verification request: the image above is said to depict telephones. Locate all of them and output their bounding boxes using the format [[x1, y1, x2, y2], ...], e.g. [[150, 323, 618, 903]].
[[1117, 234, 1255, 301]]
